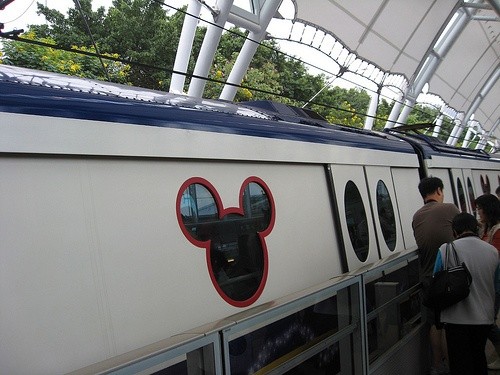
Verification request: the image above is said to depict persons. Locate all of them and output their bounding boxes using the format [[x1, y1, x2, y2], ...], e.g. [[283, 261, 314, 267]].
[[432, 213, 500, 375], [412, 171, 500, 375]]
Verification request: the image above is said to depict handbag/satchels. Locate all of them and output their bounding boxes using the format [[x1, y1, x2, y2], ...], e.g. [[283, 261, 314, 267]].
[[432, 241, 473, 306]]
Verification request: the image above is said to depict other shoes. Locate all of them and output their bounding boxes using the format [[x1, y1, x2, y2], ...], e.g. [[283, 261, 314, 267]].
[[430, 365, 449, 375], [487, 358, 500, 370]]
